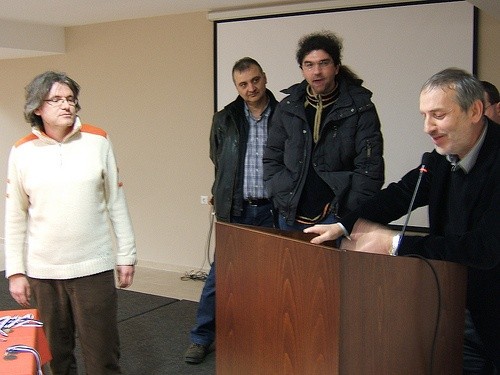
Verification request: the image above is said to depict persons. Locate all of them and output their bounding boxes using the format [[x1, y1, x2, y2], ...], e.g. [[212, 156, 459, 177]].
[[478, 81, 500, 123], [300, 67, 500, 375], [4, 70, 140, 375], [183, 56, 281, 365], [263, 30, 386, 236]]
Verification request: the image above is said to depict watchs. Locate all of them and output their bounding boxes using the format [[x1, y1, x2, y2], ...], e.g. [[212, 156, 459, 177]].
[[389, 232, 402, 256]]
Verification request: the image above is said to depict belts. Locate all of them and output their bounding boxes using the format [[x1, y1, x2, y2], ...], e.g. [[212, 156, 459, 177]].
[[245, 199, 266, 207]]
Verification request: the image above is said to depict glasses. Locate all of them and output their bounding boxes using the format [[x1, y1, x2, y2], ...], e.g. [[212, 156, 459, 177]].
[[301, 59, 333, 71], [42, 95, 79, 107]]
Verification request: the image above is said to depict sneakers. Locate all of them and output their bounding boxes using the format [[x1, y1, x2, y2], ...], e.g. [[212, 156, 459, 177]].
[[183, 343, 209, 362]]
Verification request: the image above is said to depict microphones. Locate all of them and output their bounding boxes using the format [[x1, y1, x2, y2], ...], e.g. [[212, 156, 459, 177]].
[[394, 152, 433, 257]]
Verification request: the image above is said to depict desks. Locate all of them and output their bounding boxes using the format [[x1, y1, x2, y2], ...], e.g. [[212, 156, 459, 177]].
[[0, 309, 54, 375]]
